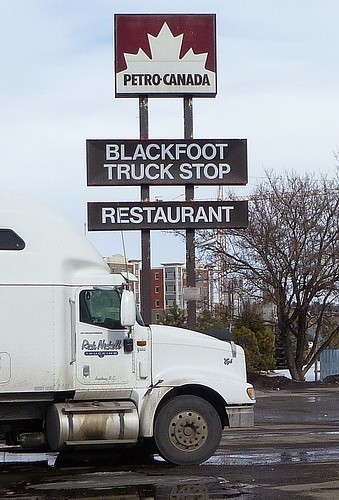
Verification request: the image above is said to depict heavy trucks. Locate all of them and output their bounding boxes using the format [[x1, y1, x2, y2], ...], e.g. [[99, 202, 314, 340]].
[[0, 223, 256, 465]]
